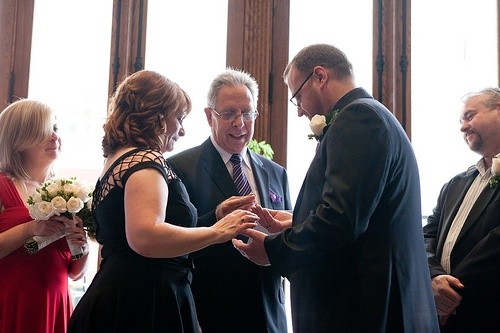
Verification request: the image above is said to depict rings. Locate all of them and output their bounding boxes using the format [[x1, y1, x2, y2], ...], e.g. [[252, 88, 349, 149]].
[[240, 216, 246, 224], [265, 225, 272, 228]]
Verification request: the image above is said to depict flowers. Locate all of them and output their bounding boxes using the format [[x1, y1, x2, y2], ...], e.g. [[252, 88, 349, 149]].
[[489, 158, 500, 188], [25, 178, 96, 259], [308, 108, 339, 142]]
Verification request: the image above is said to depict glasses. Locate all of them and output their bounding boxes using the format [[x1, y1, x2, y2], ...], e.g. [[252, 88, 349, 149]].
[[213, 109, 258, 122], [289, 63, 326, 106]]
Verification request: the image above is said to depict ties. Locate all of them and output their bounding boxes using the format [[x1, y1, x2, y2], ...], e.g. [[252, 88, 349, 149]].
[[229, 155, 256, 206]]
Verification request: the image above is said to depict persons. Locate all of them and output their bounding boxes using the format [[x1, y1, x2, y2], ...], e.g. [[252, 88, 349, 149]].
[[232, 44, 442, 333], [419, 88, 500, 333], [164, 68, 293, 333], [0, 95, 91, 333], [65, 69, 259, 332]]
